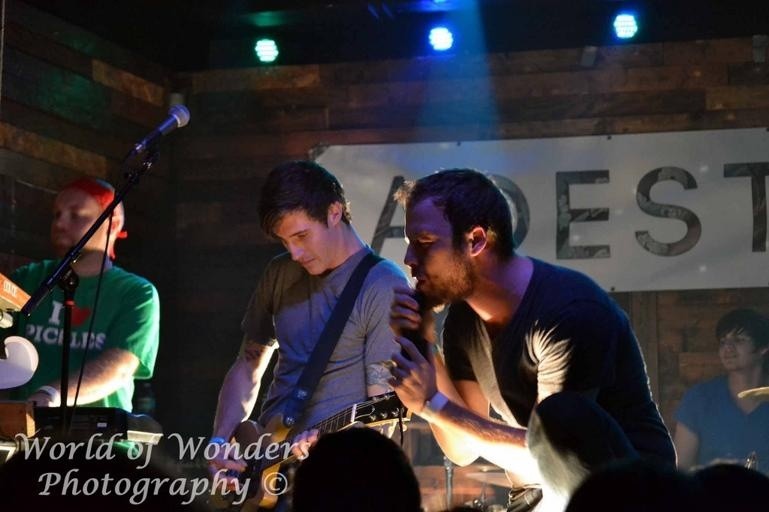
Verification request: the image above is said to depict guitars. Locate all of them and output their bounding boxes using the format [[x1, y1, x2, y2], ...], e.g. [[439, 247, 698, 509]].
[[205, 395, 412, 511]]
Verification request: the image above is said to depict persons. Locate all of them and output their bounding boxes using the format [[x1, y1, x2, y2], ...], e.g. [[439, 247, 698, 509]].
[[674, 309, 769, 478], [663, 465, 769, 512], [0, 175, 160, 456], [564, 466, 673, 512], [0, 436, 205, 512], [208, 158, 409, 512], [380, 167, 678, 511], [290, 427, 423, 512]]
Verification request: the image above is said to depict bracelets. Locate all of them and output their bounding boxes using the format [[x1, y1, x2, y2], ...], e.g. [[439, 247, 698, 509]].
[[208, 437, 228, 447], [39, 385, 59, 402], [421, 391, 450, 423]]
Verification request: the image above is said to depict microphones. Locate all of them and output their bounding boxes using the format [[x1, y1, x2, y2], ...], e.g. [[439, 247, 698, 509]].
[[401, 289, 426, 358], [132, 104, 190, 156]]
